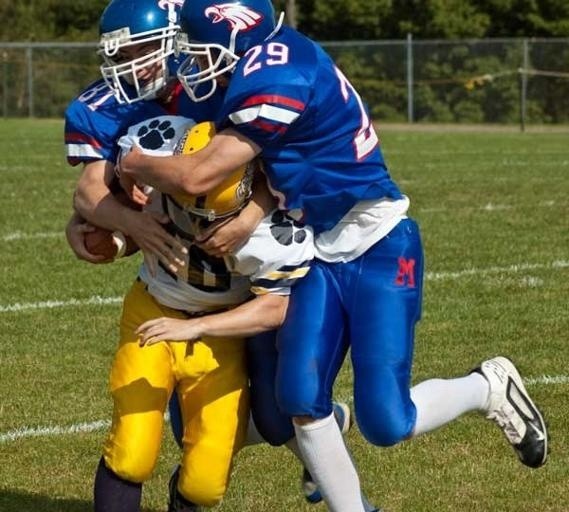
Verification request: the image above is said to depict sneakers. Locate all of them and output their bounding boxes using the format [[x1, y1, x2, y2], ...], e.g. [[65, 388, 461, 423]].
[[302, 402, 353, 502], [467, 354, 551, 468]]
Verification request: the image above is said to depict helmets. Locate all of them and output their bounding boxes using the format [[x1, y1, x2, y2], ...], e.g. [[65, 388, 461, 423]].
[[172, 1, 284, 104], [171, 121, 256, 221], [95, 1, 184, 106]]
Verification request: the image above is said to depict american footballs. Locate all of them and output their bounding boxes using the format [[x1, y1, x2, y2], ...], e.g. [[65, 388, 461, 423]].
[[84, 187, 143, 259]]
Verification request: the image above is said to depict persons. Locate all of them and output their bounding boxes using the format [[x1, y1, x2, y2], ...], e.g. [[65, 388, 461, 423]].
[[61, 0, 354, 512], [65, 0, 552, 512], [63, 117, 317, 512]]
